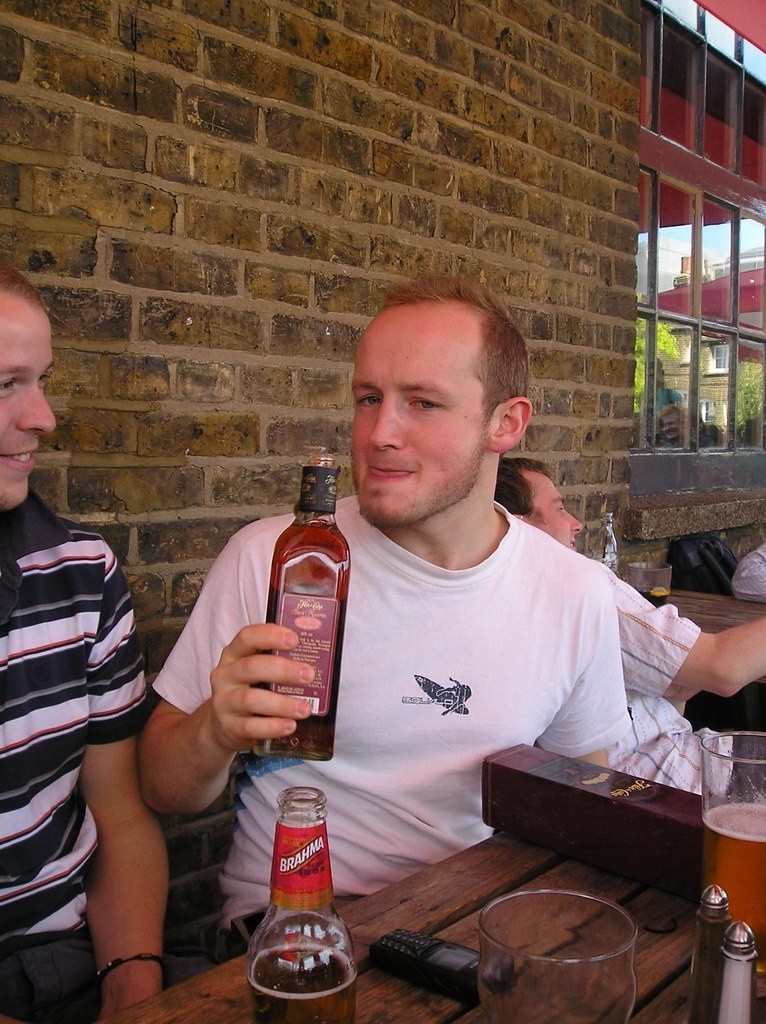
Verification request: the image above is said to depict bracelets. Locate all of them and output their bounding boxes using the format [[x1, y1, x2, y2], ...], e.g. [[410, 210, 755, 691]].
[[95, 952, 165, 983]]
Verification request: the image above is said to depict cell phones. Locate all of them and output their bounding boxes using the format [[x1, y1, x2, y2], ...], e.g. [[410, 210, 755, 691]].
[[369, 929, 485, 1003]]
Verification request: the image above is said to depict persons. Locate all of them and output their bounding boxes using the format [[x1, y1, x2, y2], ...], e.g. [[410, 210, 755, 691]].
[[138, 274, 636, 948], [732, 543, 766, 603], [0, 265, 213, 1024], [493, 454, 766, 801]]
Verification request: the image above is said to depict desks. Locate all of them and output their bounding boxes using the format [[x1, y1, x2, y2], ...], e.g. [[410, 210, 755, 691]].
[[98, 823, 766, 1024], [657, 588, 766, 731]]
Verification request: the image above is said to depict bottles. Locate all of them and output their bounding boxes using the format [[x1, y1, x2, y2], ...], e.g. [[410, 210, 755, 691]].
[[690, 885, 734, 1024], [714, 920, 757, 1023], [247, 787, 361, 1023], [253, 454, 350, 761], [590, 512, 618, 576]]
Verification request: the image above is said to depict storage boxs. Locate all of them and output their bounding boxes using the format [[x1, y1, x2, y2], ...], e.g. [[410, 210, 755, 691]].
[[481, 743, 725, 904]]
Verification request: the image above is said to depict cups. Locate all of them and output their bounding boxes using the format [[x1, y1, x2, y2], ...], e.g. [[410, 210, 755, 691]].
[[700, 732, 765, 997], [475, 889, 637, 1023], [628, 562, 672, 608]]
